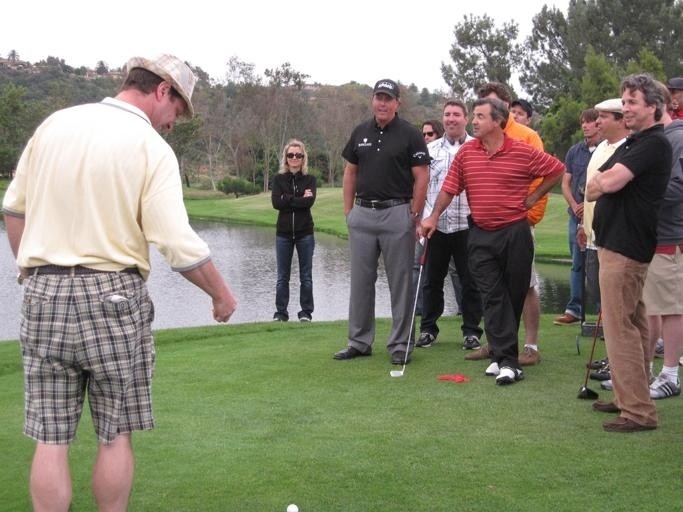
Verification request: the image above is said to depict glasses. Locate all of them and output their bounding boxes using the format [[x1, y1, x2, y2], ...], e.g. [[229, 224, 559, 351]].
[[424, 132, 434, 138], [287, 153, 303, 159]]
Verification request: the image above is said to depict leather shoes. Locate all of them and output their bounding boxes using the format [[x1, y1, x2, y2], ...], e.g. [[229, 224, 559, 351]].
[[464, 346, 491, 361], [333, 346, 372, 360], [592, 401, 618, 413], [392, 351, 410, 364], [518, 346, 540, 365], [602, 417, 656, 432]]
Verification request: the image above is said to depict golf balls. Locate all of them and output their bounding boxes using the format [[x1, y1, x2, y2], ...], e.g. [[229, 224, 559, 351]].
[[287, 503, 299, 511]]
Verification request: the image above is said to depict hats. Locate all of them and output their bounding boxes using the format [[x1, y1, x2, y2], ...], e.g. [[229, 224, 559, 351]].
[[373, 79, 400, 98], [126, 54, 195, 125], [666, 78, 683, 90], [594, 98, 623, 113]]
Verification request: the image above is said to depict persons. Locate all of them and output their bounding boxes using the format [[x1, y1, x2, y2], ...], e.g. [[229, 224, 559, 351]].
[[413, 81, 569, 384], [333, 79, 431, 365], [1, 53, 236, 512], [554, 75, 683, 433], [272, 140, 317, 321]]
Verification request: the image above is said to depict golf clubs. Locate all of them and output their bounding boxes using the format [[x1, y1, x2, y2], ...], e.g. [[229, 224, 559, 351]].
[[577, 308, 600, 399], [390, 237, 429, 378]]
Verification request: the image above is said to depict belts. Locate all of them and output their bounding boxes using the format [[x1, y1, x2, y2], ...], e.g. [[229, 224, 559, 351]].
[[354, 198, 410, 208], [656, 245, 683, 256], [22, 263, 140, 277]]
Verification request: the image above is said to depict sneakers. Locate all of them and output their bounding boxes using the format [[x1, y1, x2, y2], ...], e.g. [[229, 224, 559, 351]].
[[587, 339, 682, 398], [553, 314, 582, 325], [462, 335, 481, 350], [496, 367, 524, 385], [414, 332, 439, 347], [485, 362, 499, 375]]
